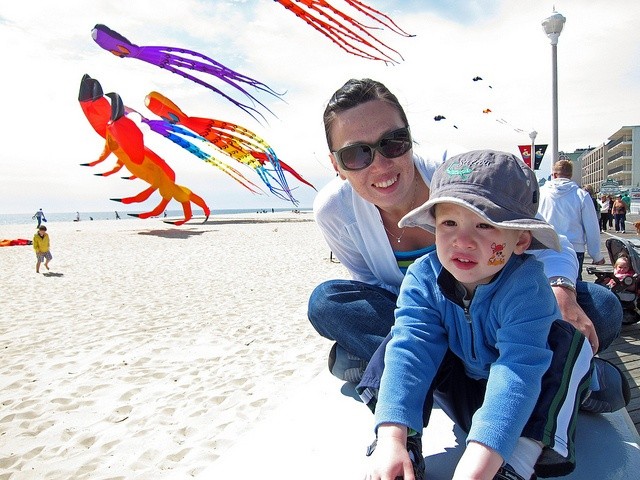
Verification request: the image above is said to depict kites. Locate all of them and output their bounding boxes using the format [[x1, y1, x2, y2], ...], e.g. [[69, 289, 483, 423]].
[[483, 109, 523, 132]]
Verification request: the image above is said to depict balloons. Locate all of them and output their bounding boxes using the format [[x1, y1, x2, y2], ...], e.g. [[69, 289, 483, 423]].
[[144, 91, 318, 207], [122, 104, 270, 198], [91, 24, 289, 126], [274, 0, 416, 67], [78, 74, 211, 226]]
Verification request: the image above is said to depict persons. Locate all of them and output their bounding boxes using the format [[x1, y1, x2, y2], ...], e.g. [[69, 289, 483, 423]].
[[598, 196, 609, 234], [584, 184, 598, 215], [90, 217, 93, 220], [607, 256, 634, 289], [115, 211, 120, 220], [621, 194, 630, 220], [164, 210, 167, 218], [534, 159, 606, 281], [606, 195, 615, 230], [308, 78, 631, 414], [257, 209, 267, 213], [272, 208, 274, 213], [34, 226, 52, 273], [77, 212, 79, 221], [33, 208, 45, 228], [611, 194, 626, 233], [355, 150, 594, 480]]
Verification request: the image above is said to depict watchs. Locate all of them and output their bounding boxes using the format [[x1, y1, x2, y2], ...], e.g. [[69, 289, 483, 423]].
[[548, 276, 577, 296]]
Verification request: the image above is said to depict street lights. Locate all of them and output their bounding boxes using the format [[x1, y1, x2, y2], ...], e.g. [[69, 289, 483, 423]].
[[541, 5, 566, 180], [529, 131, 538, 172]]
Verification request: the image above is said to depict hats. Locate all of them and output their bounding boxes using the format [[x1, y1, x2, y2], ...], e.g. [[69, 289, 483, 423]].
[[397, 150, 562, 253]]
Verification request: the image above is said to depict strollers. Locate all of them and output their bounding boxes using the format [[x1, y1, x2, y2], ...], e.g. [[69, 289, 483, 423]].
[[586, 236, 640, 325]]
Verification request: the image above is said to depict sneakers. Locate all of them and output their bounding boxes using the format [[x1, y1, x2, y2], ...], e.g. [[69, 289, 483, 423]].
[[365, 434, 426, 480], [328, 342, 369, 384], [579, 357, 631, 413]]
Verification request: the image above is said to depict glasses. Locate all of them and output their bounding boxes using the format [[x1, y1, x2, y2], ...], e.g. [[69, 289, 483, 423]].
[[332, 126, 413, 171]]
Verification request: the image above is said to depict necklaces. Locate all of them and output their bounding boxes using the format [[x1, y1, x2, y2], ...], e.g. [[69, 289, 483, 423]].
[[373, 167, 419, 243]]
